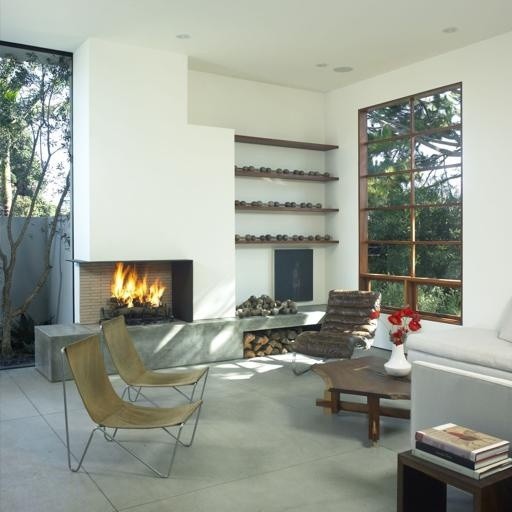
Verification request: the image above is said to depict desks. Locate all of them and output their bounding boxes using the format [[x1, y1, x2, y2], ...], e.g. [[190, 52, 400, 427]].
[[396, 450, 512, 512], [308, 357, 413, 448]]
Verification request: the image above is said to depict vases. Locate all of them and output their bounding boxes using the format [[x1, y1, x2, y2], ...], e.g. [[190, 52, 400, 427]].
[[383, 342, 412, 378]]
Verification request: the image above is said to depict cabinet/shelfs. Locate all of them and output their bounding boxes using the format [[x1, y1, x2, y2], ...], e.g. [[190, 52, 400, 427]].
[[234, 135, 340, 247]]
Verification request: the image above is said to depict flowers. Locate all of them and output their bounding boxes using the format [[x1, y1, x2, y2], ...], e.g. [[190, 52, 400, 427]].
[[386, 307, 423, 346]]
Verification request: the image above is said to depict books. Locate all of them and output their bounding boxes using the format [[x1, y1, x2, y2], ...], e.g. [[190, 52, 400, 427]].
[[412, 422, 512, 481]]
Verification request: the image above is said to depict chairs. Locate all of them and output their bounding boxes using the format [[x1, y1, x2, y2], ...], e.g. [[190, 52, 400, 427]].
[[291, 290, 382, 376], [58, 315, 210, 478]]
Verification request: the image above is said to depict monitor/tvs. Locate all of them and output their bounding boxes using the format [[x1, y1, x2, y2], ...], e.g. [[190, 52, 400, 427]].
[[272, 247, 313, 302]]
